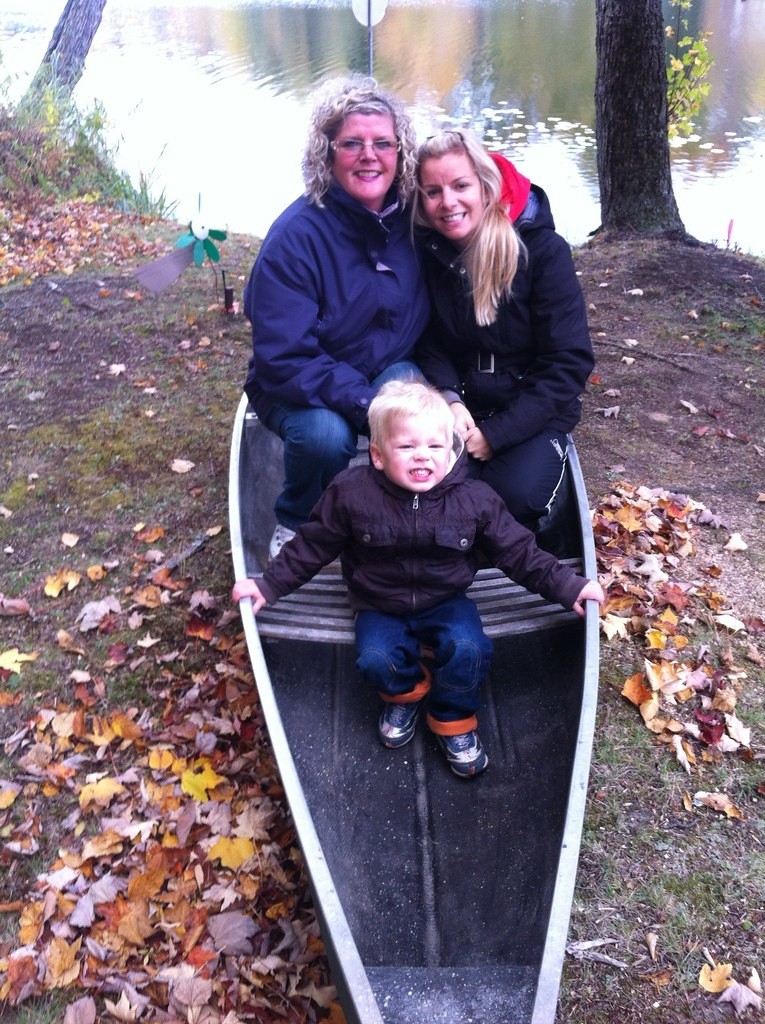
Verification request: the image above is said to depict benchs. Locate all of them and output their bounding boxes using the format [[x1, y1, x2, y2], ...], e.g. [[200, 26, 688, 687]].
[[250, 558, 583, 645]]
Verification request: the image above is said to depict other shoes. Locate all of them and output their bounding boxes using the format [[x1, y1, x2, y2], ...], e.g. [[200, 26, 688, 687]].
[[268, 523, 295, 561]]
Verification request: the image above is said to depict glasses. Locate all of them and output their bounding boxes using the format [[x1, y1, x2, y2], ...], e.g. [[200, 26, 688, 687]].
[[329, 139, 402, 157]]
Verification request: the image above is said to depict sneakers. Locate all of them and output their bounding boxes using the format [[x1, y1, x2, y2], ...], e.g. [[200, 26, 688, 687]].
[[377, 702, 420, 749], [436, 729, 489, 778]]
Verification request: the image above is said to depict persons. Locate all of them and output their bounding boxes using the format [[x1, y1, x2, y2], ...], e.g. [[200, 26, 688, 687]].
[[243, 74, 431, 562], [232, 380, 605, 778], [411, 129, 596, 545]]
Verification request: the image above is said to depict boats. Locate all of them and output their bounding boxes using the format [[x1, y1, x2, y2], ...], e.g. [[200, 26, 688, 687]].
[[226, 389, 601, 1024]]
